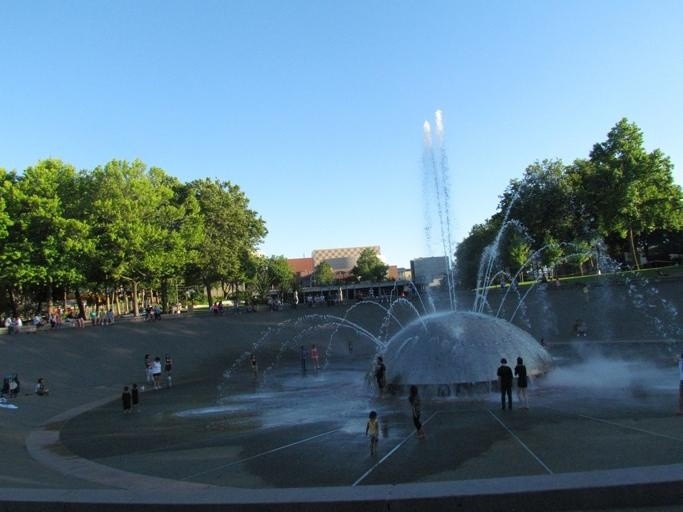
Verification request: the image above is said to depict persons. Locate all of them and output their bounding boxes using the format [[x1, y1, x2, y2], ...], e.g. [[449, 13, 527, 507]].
[[34, 377, 49, 395], [364, 409, 381, 459], [496, 356, 514, 410], [9, 370, 20, 397], [407, 385, 426, 438], [678, 358, 683, 414], [542, 274, 562, 288], [514, 355, 529, 408], [300, 344, 310, 367], [0, 304, 114, 334], [249, 352, 259, 375], [311, 344, 319, 372], [145, 305, 161, 320], [210, 288, 375, 315], [373, 355, 388, 398], [122, 353, 174, 414]]
